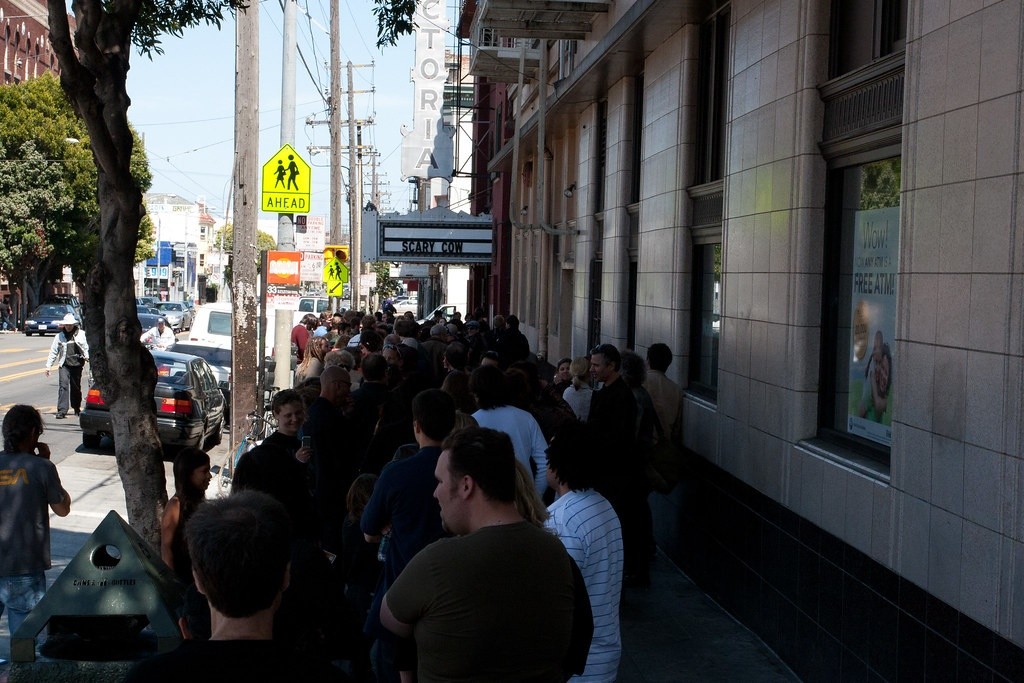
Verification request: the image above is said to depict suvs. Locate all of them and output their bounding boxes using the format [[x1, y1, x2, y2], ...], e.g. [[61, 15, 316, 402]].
[[42, 294, 80, 315]]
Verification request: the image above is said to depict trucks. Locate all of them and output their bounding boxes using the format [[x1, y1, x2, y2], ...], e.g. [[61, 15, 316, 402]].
[[415, 304, 456, 327]]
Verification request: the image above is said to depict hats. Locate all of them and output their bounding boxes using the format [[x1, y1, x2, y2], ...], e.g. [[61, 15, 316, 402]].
[[493, 315, 505, 328], [402, 337, 418, 350], [384, 334, 400, 344], [404, 311, 414, 321], [430, 325, 447, 335], [466, 320, 480, 328], [360, 331, 383, 353], [59, 313, 78, 324]]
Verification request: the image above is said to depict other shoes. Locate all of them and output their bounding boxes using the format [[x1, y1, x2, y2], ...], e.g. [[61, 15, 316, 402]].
[[56, 412, 65, 419], [74, 408, 81, 416]]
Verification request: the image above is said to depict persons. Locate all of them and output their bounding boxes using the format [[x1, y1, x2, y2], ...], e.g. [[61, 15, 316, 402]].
[[45, 313, 90, 419], [857, 332, 892, 426], [140, 317, 175, 352], [0, 405, 71, 667], [0, 299, 18, 333], [119, 307, 688, 683]]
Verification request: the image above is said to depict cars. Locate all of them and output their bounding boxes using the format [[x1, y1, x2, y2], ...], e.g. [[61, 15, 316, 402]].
[[168, 341, 233, 403], [391, 297, 418, 314], [23, 304, 81, 336], [137, 313, 171, 336], [78, 351, 229, 453], [153, 302, 192, 332], [134, 296, 160, 315]]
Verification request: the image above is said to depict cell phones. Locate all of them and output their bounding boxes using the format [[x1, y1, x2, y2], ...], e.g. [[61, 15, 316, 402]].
[[302, 436, 311, 446]]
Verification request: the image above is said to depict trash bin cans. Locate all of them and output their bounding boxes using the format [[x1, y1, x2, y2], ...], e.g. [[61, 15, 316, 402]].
[[360, 295, 367, 308], [7, 510, 197, 683], [400, 288, 403, 296]]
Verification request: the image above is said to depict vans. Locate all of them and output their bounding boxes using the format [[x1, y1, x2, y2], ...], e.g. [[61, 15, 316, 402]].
[[293, 296, 329, 325], [190, 301, 273, 384]]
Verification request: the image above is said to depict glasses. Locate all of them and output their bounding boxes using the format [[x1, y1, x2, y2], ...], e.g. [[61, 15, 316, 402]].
[[332, 380, 352, 387], [358, 345, 364, 349], [383, 345, 402, 357], [158, 322, 163, 325], [35, 431, 42, 434], [338, 363, 352, 372], [466, 326, 475, 331], [596, 344, 604, 351]]
